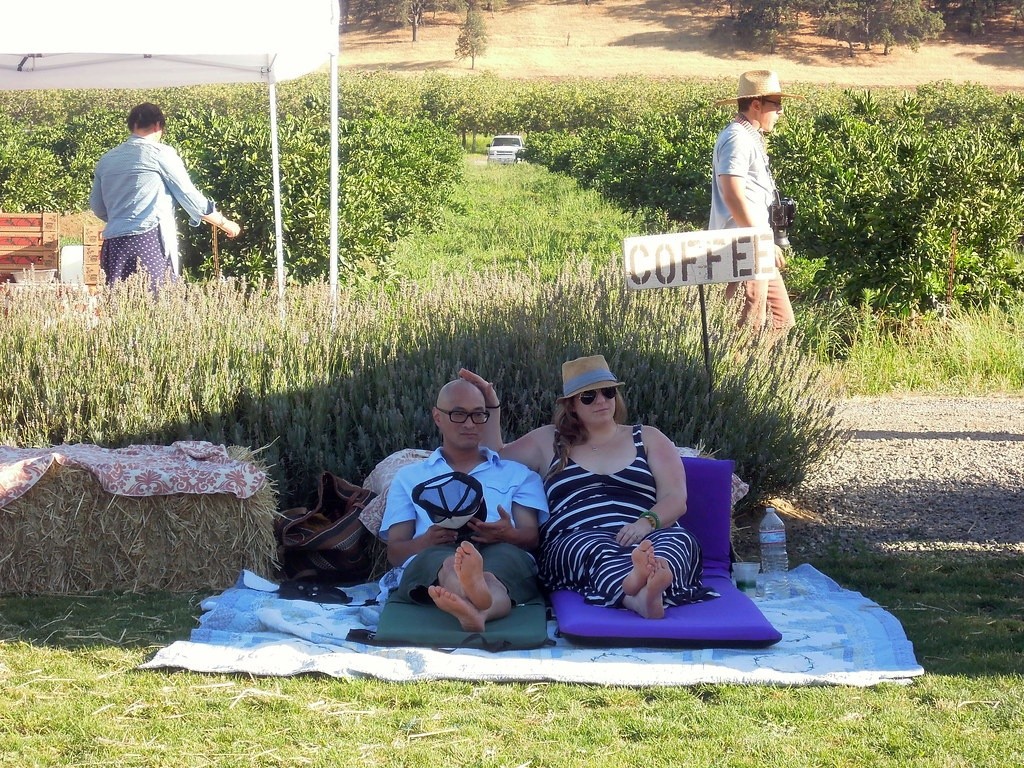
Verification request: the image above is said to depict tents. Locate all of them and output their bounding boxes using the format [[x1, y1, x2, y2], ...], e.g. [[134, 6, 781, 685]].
[[0, 0, 338, 330]]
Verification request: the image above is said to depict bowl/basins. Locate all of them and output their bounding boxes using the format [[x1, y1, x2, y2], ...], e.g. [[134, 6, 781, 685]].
[[10, 269, 57, 284]]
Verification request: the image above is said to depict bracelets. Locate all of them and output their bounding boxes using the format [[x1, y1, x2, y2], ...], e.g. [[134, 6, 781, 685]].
[[485, 401, 501, 408], [639, 512, 660, 532]]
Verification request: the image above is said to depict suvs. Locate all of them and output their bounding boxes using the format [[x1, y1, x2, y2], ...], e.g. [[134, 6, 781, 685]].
[[485, 134, 526, 164]]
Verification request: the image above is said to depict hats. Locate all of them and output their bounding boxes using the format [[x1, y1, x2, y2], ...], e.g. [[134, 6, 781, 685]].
[[556, 354, 626, 404], [715, 70, 804, 105], [412, 471, 487, 536]]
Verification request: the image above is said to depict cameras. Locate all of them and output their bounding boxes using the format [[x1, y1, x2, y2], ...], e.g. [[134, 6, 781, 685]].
[[772, 197, 799, 250]]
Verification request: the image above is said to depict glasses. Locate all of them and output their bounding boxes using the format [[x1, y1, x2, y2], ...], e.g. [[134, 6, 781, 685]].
[[760, 99, 781, 108], [436, 407, 491, 424], [572, 386, 616, 405]]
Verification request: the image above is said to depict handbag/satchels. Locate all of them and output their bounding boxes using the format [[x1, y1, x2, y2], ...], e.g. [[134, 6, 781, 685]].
[[273, 472, 379, 572]]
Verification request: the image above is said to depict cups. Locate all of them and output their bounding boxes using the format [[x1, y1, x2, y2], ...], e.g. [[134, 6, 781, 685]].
[[731, 562, 760, 600]]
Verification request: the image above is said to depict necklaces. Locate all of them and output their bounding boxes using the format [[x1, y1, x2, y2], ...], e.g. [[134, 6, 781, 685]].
[[588, 423, 618, 451]]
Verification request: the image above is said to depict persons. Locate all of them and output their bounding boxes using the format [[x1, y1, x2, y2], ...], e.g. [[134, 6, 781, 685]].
[[459, 354, 722, 619], [379, 379, 550, 632], [90, 102, 240, 298], [709, 71, 804, 332]]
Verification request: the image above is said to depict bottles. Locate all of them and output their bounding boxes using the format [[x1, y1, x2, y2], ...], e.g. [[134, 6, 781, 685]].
[[759, 507, 791, 602]]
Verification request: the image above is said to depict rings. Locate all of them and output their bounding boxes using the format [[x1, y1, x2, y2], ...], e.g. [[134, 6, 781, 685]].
[[634, 534, 639, 539]]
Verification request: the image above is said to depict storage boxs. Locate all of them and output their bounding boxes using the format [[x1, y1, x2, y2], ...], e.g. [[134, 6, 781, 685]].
[[0, 212, 105, 285]]
